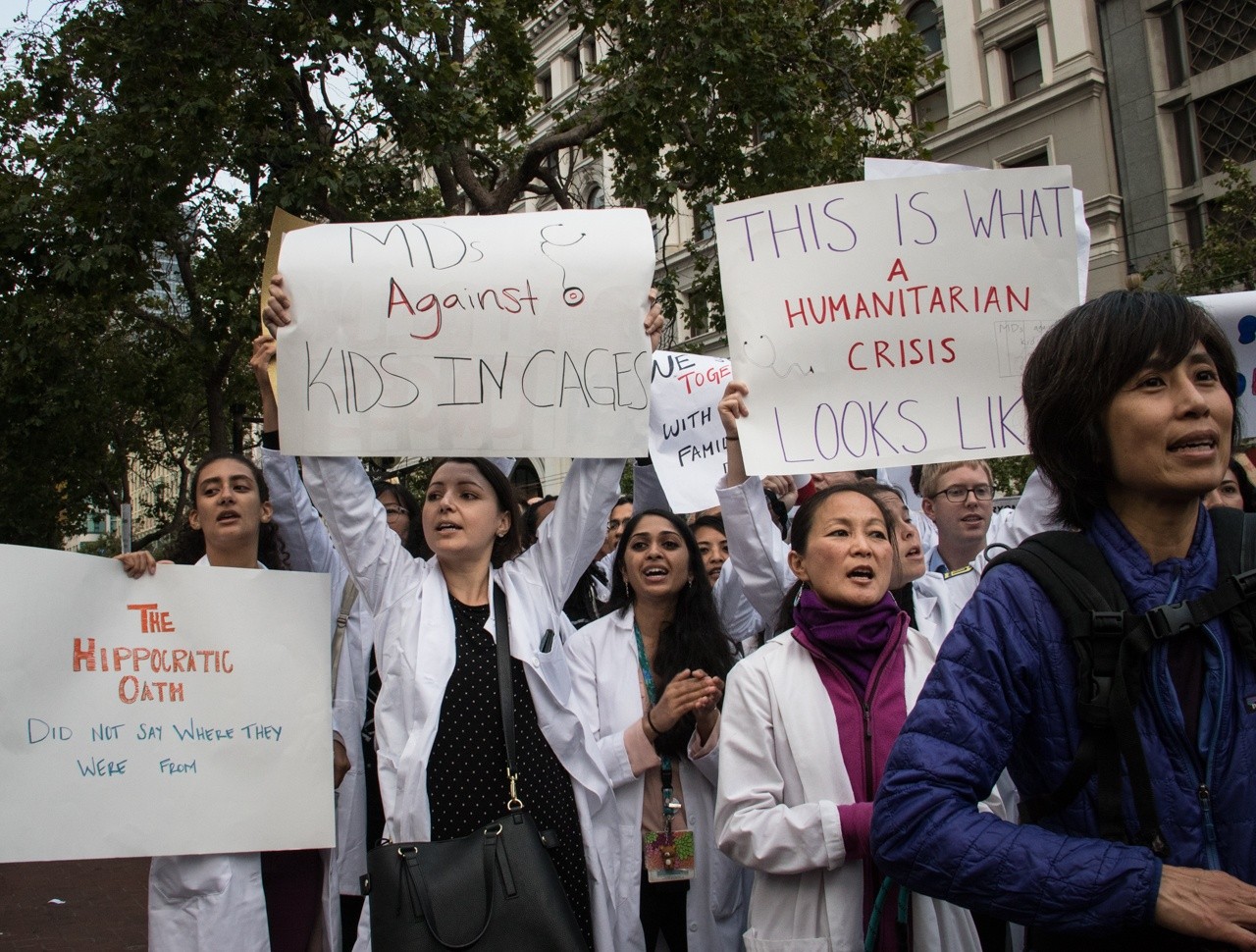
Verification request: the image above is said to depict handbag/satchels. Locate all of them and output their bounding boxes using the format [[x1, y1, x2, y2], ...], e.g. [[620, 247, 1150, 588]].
[[357, 799, 591, 952]]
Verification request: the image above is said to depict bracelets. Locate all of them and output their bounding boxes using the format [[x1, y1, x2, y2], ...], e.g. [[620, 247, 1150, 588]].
[[647, 711, 664, 735], [726, 437, 739, 440]]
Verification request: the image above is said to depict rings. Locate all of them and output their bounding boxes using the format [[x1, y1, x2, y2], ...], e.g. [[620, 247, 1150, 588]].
[[714, 704, 717, 710]]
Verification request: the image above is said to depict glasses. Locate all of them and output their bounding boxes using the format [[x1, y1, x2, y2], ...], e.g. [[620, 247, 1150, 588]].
[[928, 484, 997, 503], [606, 519, 630, 530], [384, 503, 410, 523]]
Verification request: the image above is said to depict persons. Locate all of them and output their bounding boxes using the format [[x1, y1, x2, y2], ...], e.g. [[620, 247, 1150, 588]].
[[1201, 452, 1256, 514], [864, 288, 1256, 952], [113, 273, 1081, 952]]
